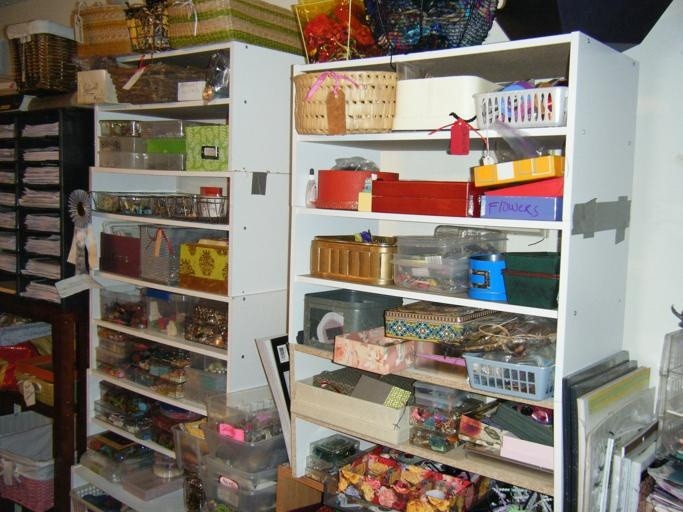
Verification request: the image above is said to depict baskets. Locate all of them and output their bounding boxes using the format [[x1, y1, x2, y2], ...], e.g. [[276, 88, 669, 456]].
[[475, 86, 567, 129], [0, 421, 55, 512], [0, 1, 305, 104], [68, 482, 129, 512], [288, 71, 397, 135], [364, 0, 496, 54], [5, 0, 305, 104], [461, 349, 553, 402]]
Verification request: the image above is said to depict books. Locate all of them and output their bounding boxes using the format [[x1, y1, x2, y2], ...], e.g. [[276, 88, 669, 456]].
[[560, 350, 681, 511]]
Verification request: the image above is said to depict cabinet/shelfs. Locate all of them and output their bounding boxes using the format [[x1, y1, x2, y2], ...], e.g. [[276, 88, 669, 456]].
[[72, 40, 304, 511], [0, 100, 91, 510], [290, 35, 639, 511]]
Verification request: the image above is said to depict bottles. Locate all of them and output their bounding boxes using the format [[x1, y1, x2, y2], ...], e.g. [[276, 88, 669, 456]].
[[198, 186, 223, 219], [306, 166, 317, 209]]
